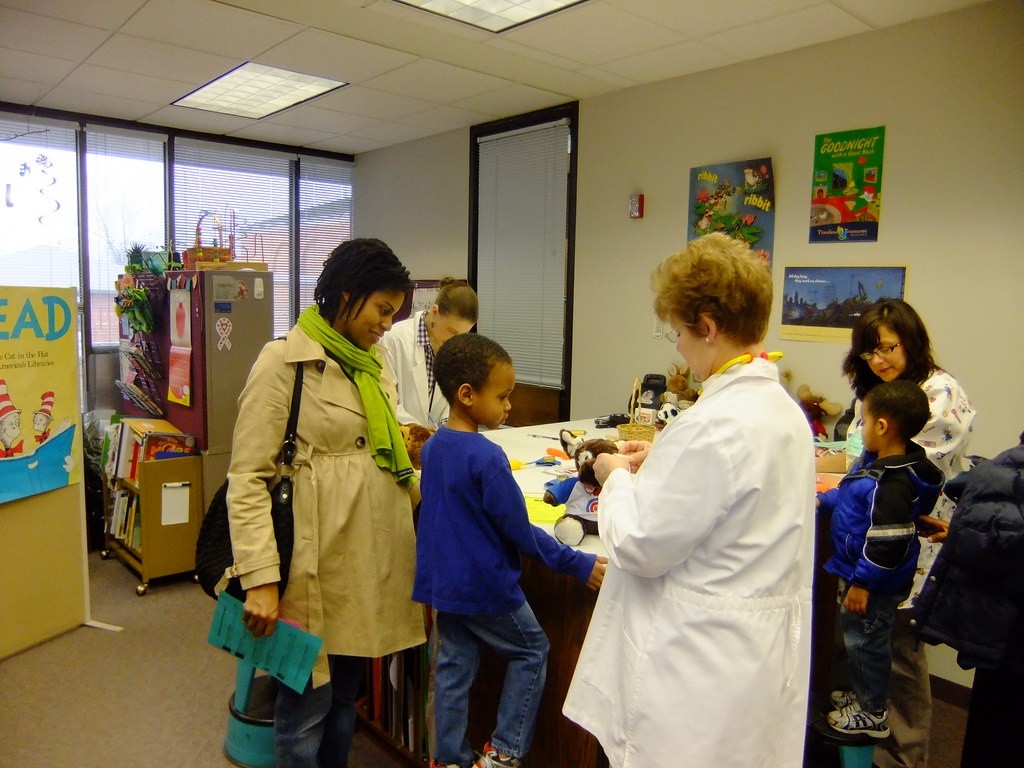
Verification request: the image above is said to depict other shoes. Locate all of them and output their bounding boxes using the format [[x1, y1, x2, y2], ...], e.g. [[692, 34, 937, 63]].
[[472, 742, 522, 768], [430, 759, 462, 768]]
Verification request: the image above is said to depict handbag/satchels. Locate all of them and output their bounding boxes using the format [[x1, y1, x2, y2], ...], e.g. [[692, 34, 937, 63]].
[[195, 477, 294, 603]]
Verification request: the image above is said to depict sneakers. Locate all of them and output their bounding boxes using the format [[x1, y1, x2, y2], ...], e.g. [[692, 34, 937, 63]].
[[830, 690, 891, 717], [827, 699, 891, 738]]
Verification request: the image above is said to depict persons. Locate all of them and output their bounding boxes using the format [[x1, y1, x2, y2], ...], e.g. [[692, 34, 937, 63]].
[[908, 435, 1024, 768], [562, 230, 816, 768], [841, 298, 977, 768], [214, 237, 427, 768], [373, 276, 479, 435], [808, 380, 946, 739], [409, 332, 607, 768]]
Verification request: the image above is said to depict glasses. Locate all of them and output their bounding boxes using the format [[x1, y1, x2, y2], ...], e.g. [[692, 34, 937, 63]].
[[664, 320, 688, 343], [860, 342, 902, 360]]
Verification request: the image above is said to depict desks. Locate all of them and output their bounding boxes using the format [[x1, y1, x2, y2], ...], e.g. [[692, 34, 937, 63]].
[[349, 412, 849, 768]]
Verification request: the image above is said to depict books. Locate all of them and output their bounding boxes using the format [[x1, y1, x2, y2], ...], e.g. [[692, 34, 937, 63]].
[[82, 346, 198, 553]]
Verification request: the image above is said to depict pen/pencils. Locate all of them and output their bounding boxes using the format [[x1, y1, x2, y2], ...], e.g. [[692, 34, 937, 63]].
[[527, 433, 560, 440], [596, 425, 615, 428]]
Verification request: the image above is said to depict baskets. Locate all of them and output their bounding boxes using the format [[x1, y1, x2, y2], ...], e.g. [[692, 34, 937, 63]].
[[187, 213, 230, 270], [616, 378, 656, 443]]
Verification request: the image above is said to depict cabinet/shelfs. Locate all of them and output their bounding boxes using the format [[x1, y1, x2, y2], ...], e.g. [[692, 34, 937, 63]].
[[100, 270, 274, 594]]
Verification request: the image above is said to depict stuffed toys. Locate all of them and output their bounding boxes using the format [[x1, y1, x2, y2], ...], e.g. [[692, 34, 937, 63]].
[[783, 367, 855, 445], [543, 429, 618, 547], [654, 362, 700, 433]]
